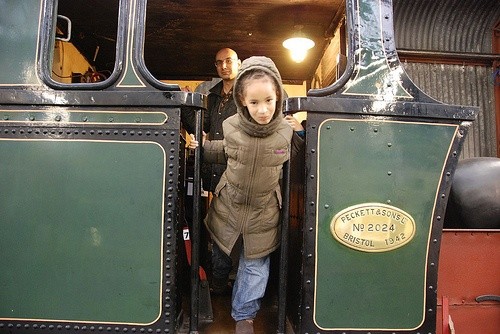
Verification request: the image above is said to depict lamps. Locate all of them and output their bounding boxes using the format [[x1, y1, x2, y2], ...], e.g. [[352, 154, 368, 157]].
[[280, 24, 315, 62]]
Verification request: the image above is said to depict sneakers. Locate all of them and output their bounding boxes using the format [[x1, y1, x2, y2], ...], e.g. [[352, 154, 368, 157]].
[[212, 273, 229, 295], [235, 320, 254, 334]]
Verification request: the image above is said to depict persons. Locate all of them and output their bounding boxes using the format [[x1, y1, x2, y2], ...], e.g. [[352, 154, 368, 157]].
[[203, 47, 242, 192], [189, 56, 304, 333]]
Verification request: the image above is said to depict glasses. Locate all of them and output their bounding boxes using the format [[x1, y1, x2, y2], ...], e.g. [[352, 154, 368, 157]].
[[215, 59, 238, 67]]
